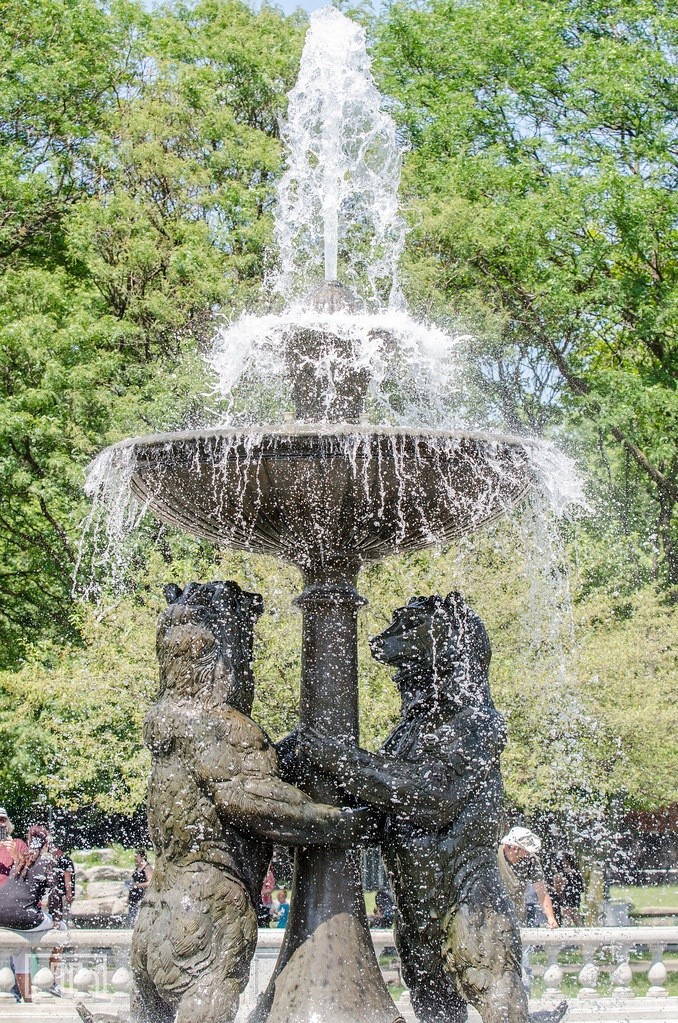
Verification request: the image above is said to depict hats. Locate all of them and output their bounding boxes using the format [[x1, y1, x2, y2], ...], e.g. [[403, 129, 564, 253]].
[[501, 827, 541, 853]]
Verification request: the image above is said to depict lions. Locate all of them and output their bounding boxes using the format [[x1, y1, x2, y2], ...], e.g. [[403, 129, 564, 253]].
[[127, 580, 528, 1022]]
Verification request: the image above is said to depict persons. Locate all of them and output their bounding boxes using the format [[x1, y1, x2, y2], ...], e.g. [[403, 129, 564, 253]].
[[128, 848, 154, 930], [366, 892, 396, 929], [497, 826, 584, 929], [261, 860, 290, 928], [0, 808, 76, 1003]]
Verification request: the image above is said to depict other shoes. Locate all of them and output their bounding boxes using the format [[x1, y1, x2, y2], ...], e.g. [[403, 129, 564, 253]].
[[49, 985, 64, 997]]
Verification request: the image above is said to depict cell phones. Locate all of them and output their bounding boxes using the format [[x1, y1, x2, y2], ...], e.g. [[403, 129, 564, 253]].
[[0, 826, 7, 843]]
